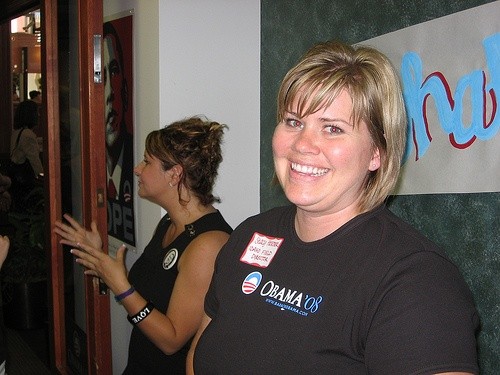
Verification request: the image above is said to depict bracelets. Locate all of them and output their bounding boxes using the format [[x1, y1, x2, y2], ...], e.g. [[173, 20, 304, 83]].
[[113, 286, 135, 302], [126, 303, 154, 325]]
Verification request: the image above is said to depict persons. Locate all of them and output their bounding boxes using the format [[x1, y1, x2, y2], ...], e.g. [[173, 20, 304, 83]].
[[52, 116, 235, 375], [0, 153, 53, 375], [28, 90, 41, 102], [8, 100, 44, 179], [183, 41, 481, 375]]
